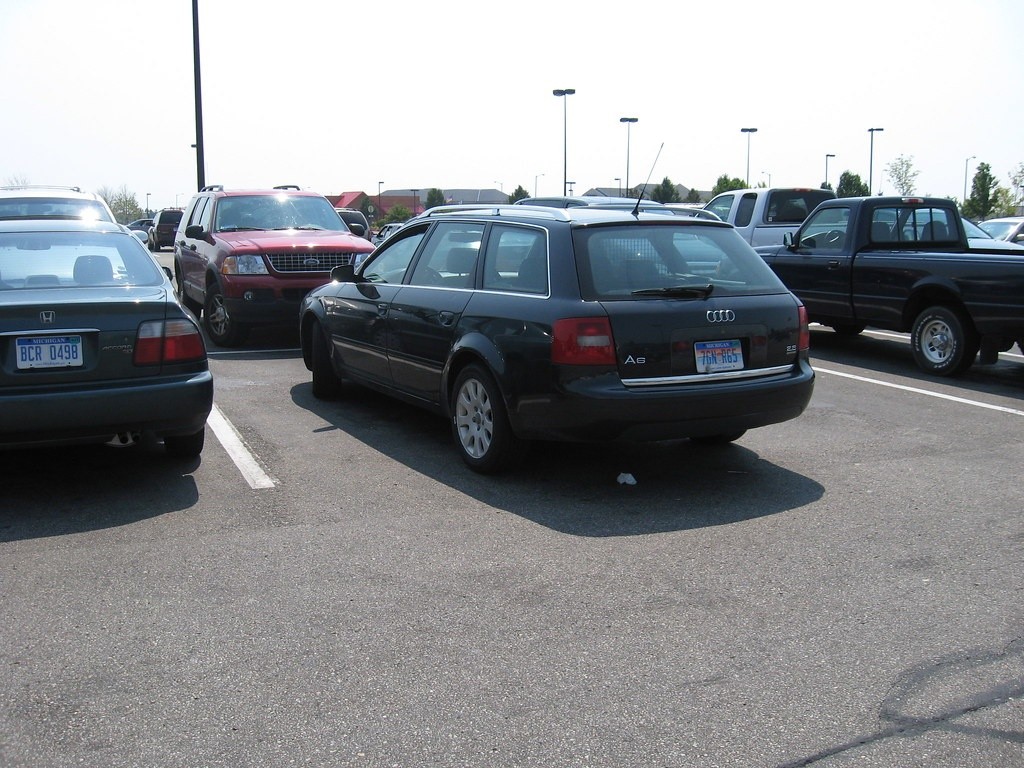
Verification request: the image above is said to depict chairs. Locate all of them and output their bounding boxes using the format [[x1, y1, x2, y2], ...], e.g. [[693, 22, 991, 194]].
[[920, 221, 947, 240], [518, 260, 540, 282], [73, 255, 112, 284], [872, 222, 891, 241], [445, 247, 478, 285]]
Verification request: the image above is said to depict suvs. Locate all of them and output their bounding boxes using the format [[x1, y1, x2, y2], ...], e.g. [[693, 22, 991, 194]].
[[145, 208, 184, 252], [298, 197, 816, 482], [333, 207, 376, 248], [173, 184, 378, 348]]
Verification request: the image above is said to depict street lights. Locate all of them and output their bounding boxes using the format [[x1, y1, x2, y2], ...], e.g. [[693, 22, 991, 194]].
[[760, 171, 773, 187], [378, 181, 384, 231], [615, 178, 622, 197], [620, 117, 639, 197], [493, 179, 505, 191], [866, 128, 883, 196], [146, 192, 152, 219], [552, 88, 576, 199], [741, 127, 758, 189], [963, 154, 976, 208], [565, 181, 576, 196], [824, 154, 836, 189], [411, 188, 419, 216], [534, 174, 545, 198]]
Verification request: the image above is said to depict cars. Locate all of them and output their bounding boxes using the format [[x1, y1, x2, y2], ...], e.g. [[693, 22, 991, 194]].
[[126, 219, 154, 244], [843, 209, 1024, 250], [371, 223, 404, 249], [976, 215, 1024, 249], [0, 185, 118, 224], [503, 196, 730, 221], [1, 219, 214, 463]]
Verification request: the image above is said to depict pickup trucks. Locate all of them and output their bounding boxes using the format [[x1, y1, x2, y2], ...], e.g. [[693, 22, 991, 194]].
[[688, 186, 929, 268], [763, 196, 1024, 378]]
